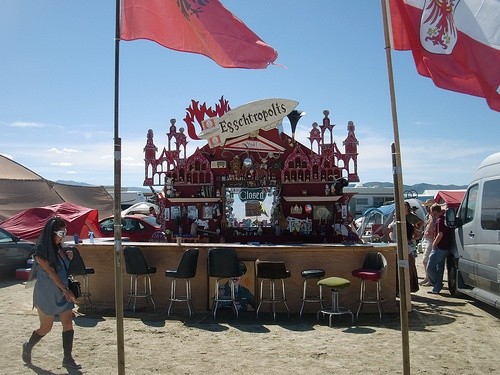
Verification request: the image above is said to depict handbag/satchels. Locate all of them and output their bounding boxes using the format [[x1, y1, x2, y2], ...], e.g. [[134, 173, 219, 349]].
[[67, 278, 82, 298]]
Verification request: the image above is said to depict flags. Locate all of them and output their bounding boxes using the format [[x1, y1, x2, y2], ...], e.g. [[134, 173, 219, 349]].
[[386, 0, 500, 112], [119, 0, 279, 69]]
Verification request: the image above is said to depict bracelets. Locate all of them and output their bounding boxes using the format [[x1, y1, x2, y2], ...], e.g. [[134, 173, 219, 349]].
[[62, 287, 70, 290]]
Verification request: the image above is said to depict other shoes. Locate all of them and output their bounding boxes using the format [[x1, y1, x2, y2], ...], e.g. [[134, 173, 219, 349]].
[[419, 278, 434, 286], [62, 356, 81, 369], [23, 342, 31, 363]]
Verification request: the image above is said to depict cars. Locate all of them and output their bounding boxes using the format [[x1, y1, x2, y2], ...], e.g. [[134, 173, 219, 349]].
[[97, 214, 161, 242], [0, 228, 37, 274]]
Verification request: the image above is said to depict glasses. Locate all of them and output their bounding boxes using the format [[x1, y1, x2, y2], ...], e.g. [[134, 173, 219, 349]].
[[54, 227, 67, 238]]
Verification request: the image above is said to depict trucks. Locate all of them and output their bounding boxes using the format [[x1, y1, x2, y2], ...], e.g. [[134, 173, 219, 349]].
[[445, 152, 500, 310]]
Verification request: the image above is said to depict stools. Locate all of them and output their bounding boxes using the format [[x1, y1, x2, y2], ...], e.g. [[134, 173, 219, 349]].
[[255, 259, 291, 323], [351, 253, 387, 323], [64, 246, 94, 314], [299, 269, 326, 320], [123, 246, 156, 314], [208, 247, 246, 321], [165, 249, 200, 317], [317, 276, 353, 327]]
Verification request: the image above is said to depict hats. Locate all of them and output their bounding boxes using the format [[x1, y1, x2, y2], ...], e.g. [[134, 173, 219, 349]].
[[421, 199, 434, 205], [411, 206, 419, 209]]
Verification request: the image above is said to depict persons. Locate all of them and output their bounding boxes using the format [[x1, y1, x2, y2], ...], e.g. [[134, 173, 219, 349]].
[[382, 199, 452, 294], [22, 218, 82, 369]]
